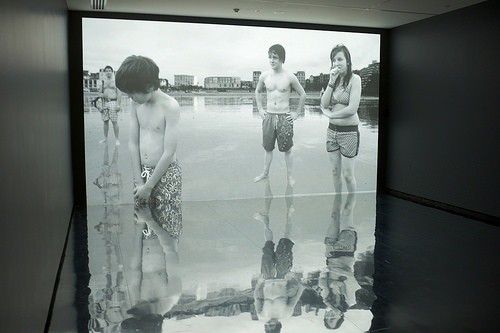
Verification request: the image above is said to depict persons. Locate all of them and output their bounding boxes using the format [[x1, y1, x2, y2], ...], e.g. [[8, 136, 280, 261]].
[[318, 193, 357, 329], [254, 44, 306, 188], [252, 204, 305, 333], [96, 206, 128, 333], [321, 46, 361, 192], [115, 55, 180, 202], [120, 203, 186, 333], [98, 65, 121, 146]]
